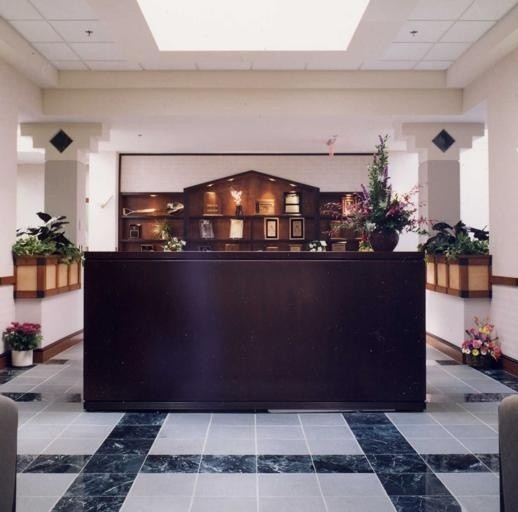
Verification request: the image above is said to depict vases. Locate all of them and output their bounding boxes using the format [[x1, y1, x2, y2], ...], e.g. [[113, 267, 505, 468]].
[[12, 350, 34, 367], [370, 231, 398, 252], [466, 354, 494, 369]]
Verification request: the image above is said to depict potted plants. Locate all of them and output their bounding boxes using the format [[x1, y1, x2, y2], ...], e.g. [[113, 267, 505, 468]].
[[13, 213, 82, 298], [421, 220, 491, 298]]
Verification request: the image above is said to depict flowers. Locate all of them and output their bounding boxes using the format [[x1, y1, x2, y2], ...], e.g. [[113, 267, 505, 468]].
[[3, 322, 43, 350], [320, 134, 434, 240], [462, 317, 502, 360]]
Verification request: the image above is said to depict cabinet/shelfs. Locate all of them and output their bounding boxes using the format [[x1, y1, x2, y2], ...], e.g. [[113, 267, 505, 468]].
[[119, 170, 364, 251]]
